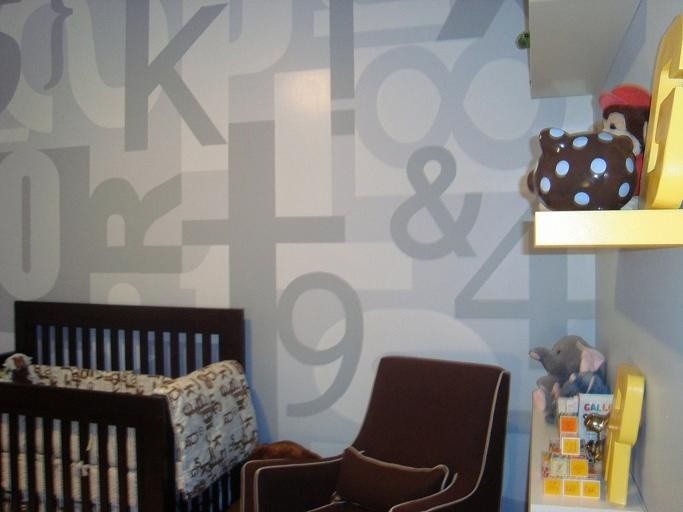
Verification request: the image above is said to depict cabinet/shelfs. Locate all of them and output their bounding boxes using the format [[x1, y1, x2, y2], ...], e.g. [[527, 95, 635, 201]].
[[522, 0, 683, 512]]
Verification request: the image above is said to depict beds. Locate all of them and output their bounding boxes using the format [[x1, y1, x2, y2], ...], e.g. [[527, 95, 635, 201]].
[[1, 300, 261, 512]]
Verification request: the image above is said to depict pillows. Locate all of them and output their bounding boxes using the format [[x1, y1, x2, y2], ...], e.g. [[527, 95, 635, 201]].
[[334, 444, 450, 511]]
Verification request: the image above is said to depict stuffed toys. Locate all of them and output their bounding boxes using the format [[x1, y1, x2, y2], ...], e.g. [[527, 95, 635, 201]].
[[528, 333, 613, 423], [596, 84, 653, 197]]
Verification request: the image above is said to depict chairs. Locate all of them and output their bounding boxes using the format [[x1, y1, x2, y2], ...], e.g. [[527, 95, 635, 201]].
[[236, 352, 513, 510]]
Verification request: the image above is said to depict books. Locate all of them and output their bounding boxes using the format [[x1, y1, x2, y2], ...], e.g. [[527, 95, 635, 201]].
[[557, 392, 613, 463]]
